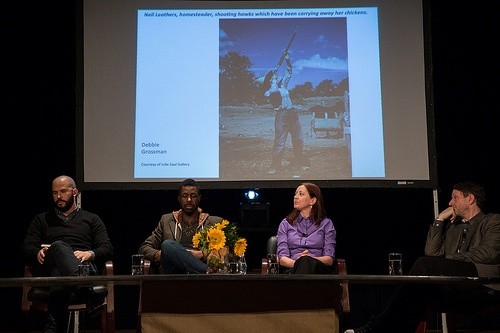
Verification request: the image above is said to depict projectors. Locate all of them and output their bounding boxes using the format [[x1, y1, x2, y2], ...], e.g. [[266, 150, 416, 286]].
[[241, 190, 264, 205]]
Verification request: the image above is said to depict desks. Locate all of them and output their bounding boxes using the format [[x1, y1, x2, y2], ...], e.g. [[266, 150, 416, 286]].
[[1, 274, 485, 286], [141, 280, 344, 333]]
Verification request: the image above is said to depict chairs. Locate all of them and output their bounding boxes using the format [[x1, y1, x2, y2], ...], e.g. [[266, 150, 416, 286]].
[[262, 236, 350, 313], [22, 260, 116, 333]]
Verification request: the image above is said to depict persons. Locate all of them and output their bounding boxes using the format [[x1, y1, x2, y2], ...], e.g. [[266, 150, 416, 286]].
[[24, 176, 114, 333], [137, 179, 224, 274], [263, 51, 311, 174], [277, 183, 336, 275], [344, 181, 500, 333]]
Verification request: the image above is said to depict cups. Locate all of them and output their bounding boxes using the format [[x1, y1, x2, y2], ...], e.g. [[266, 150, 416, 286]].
[[78, 259, 91, 277], [132, 254, 144, 274], [267, 254, 280, 274], [388, 252, 402, 275]]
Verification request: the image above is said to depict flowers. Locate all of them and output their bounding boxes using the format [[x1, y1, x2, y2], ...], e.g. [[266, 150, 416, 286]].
[[192, 220, 247, 270]]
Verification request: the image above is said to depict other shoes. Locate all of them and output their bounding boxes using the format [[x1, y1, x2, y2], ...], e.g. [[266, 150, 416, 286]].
[[72, 285, 108, 299]]
